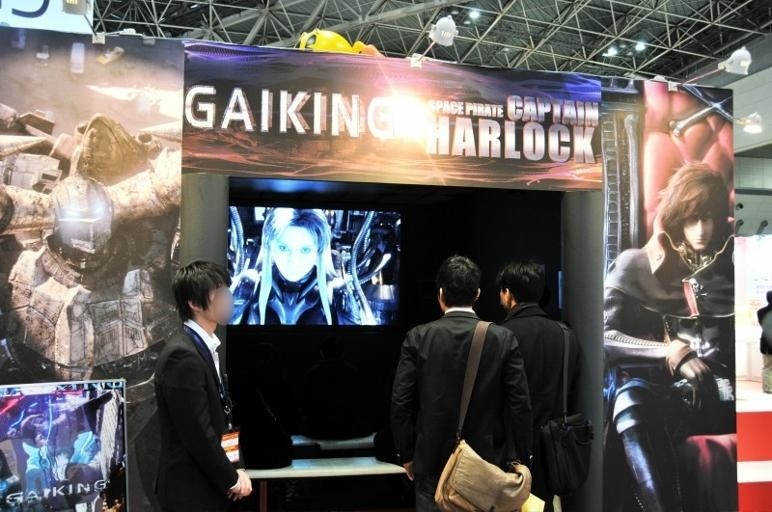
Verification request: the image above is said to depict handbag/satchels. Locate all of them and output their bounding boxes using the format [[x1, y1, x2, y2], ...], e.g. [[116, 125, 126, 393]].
[[543, 410, 594, 489], [433, 439, 533, 512]]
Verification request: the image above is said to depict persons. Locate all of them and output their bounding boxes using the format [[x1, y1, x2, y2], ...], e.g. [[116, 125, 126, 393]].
[[154, 259, 254, 512], [231, 207, 360, 324], [389, 257, 529, 511], [493, 258, 581, 512]]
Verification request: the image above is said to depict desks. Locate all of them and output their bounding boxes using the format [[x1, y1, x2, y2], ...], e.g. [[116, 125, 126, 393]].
[[229, 455, 414, 511]]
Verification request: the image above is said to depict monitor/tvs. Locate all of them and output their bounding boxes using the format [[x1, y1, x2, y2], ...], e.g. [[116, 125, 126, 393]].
[[228, 194, 412, 344]]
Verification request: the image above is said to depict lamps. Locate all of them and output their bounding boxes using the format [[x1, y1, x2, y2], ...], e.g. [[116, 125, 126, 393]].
[[405, 15, 458, 70], [732, 103, 762, 134], [682, 41, 752, 89]]
